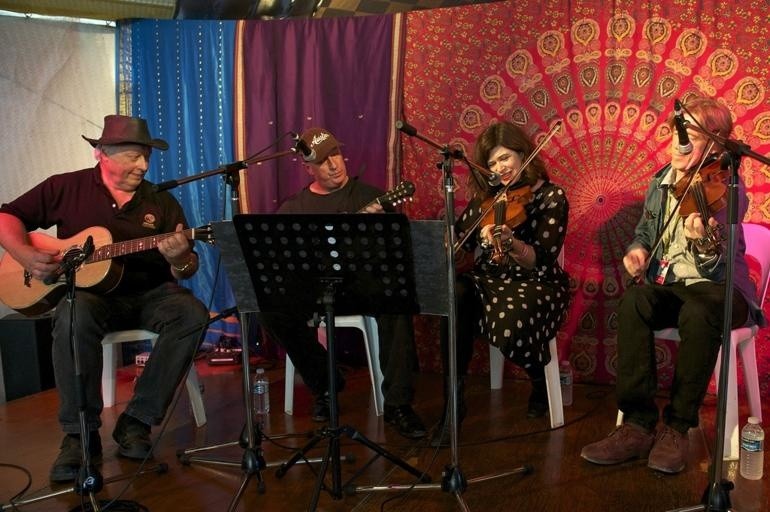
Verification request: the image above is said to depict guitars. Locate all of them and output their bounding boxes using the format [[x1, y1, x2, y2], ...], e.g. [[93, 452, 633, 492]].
[[1, 226, 214, 317]]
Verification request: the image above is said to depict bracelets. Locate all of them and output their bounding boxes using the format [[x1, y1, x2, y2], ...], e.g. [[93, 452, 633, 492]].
[[171, 259, 193, 273], [511, 240, 529, 259]]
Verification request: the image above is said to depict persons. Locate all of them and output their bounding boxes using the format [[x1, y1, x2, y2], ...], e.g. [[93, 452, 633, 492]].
[[266, 128, 429, 439], [431, 117, 575, 449], [1, 113, 211, 482], [577, 96, 764, 476]]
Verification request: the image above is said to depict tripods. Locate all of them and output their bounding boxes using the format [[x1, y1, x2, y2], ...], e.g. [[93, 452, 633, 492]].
[[1, 305, 168, 512], [177, 328, 356, 510], [268, 324, 434, 510], [344, 325, 534, 510]]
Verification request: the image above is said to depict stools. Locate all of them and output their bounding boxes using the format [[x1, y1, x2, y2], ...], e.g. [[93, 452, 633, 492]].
[[93, 328, 209, 429], [279, 314, 390, 420]]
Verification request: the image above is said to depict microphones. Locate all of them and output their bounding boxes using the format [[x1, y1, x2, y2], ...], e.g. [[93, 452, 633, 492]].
[[673, 97, 693, 155], [290, 130, 317, 162], [484, 168, 501, 187]]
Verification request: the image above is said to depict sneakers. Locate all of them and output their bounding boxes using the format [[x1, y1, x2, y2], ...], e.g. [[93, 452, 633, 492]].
[[648, 424, 690, 473], [110, 414, 153, 459], [580, 420, 656, 465], [50, 431, 103, 481], [311, 374, 346, 421], [383, 403, 427, 438]]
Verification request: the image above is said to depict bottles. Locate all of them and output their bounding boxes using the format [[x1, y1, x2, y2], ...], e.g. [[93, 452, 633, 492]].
[[252, 367, 270, 416], [740, 416, 765, 481]]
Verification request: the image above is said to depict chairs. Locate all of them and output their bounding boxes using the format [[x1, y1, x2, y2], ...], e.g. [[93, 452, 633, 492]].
[[616, 223, 770, 461], [489, 233, 566, 430]]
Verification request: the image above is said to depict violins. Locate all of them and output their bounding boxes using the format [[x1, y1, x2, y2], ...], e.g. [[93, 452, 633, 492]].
[[480, 187, 530, 267], [673, 158, 731, 249]]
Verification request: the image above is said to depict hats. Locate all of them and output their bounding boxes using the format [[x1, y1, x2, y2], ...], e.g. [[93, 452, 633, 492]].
[[81, 115, 169, 150], [300, 128, 343, 164]]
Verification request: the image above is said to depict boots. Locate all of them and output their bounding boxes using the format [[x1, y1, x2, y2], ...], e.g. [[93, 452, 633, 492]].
[[430, 377, 467, 447], [526, 368, 548, 418]]
